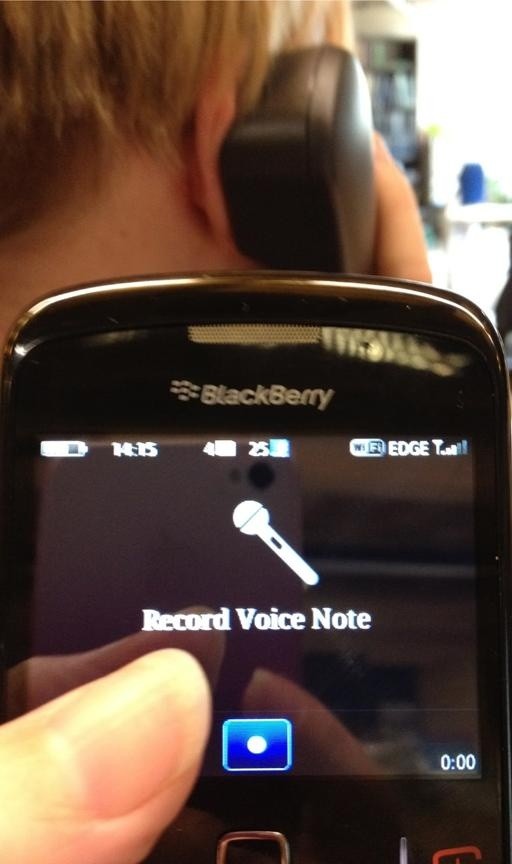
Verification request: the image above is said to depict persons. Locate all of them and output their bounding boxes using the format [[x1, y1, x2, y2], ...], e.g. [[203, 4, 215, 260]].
[[1, 644, 220, 864], [247, 669, 367, 783], [27, 604, 229, 727], [1, 0, 434, 352]]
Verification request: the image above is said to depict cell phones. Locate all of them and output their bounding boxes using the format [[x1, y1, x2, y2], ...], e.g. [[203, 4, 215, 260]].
[[0, 269, 512, 863]]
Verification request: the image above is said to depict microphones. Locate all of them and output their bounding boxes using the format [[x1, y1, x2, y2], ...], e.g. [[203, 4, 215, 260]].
[[232, 499, 320, 587]]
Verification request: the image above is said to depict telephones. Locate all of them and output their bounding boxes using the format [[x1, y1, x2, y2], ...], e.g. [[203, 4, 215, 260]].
[[217, 44, 377, 273]]
